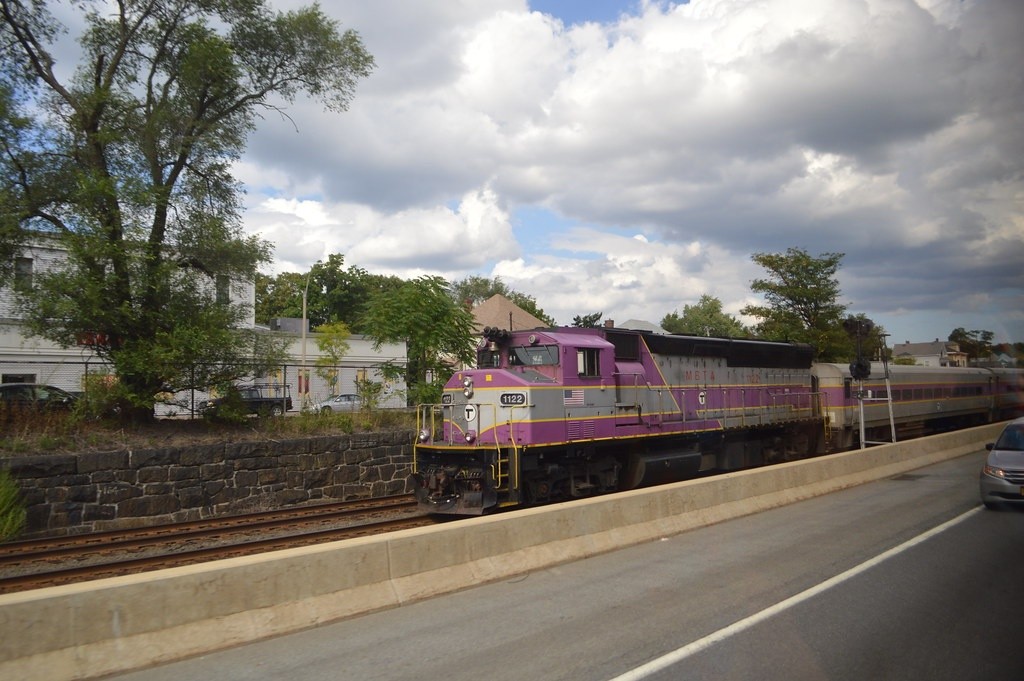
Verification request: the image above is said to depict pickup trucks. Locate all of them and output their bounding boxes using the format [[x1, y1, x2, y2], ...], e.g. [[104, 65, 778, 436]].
[[198, 382, 294, 423]]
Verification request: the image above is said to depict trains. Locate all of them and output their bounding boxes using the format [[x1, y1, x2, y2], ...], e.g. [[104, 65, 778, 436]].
[[404, 311, 1024, 515]]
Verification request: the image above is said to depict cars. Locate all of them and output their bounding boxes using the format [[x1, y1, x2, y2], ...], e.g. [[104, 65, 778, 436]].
[[308, 393, 368, 415], [979, 418, 1024, 512], [0, 382, 81, 412]]
